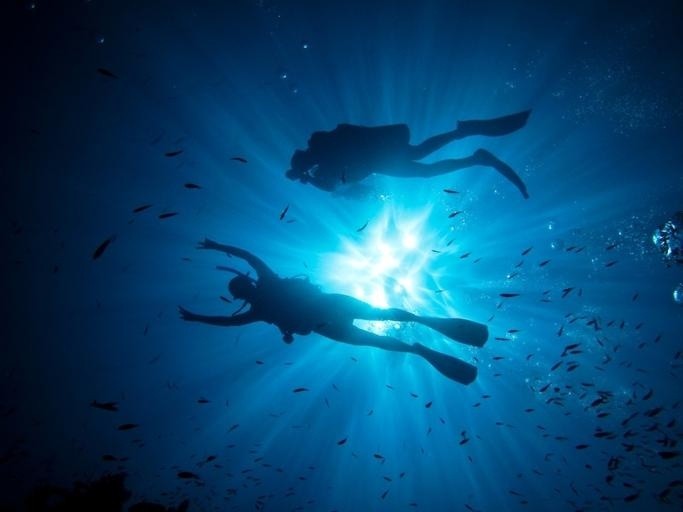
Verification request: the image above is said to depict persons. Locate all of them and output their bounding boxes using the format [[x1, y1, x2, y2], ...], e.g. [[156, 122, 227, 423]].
[[178, 239, 416, 353], [286, 123, 478, 191]]
